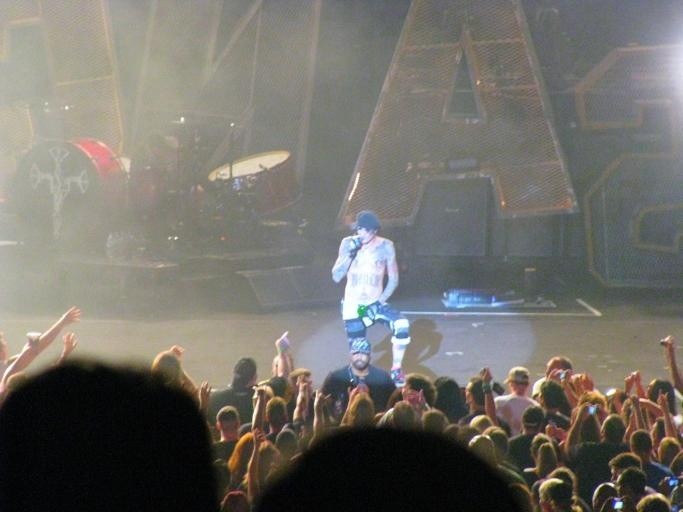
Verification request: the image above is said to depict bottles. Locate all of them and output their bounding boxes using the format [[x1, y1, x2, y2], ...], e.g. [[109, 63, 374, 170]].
[[358, 303, 375, 328]]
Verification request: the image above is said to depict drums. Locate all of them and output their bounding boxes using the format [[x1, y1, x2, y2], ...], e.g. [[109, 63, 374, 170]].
[[208, 149, 304, 218], [10, 136, 128, 243]]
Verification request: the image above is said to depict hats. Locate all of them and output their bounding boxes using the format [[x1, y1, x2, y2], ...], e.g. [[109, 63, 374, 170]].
[[355, 211, 382, 230], [503, 366, 531, 386], [233, 358, 258, 385], [349, 336, 371, 355]]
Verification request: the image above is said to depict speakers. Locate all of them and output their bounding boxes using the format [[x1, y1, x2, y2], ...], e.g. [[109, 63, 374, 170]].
[[67, 246, 345, 324], [410, 174, 495, 259]]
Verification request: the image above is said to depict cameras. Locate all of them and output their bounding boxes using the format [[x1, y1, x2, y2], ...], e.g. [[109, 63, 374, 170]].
[[668, 477, 679, 487], [610, 497, 627, 511], [560, 370, 568, 380], [584, 405, 600, 415]]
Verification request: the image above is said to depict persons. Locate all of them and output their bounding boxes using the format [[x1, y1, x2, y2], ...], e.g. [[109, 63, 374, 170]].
[[1, 305, 682, 511], [329, 210, 413, 388]]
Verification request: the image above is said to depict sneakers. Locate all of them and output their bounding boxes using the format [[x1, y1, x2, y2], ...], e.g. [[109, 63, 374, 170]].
[[390, 367, 407, 387]]
